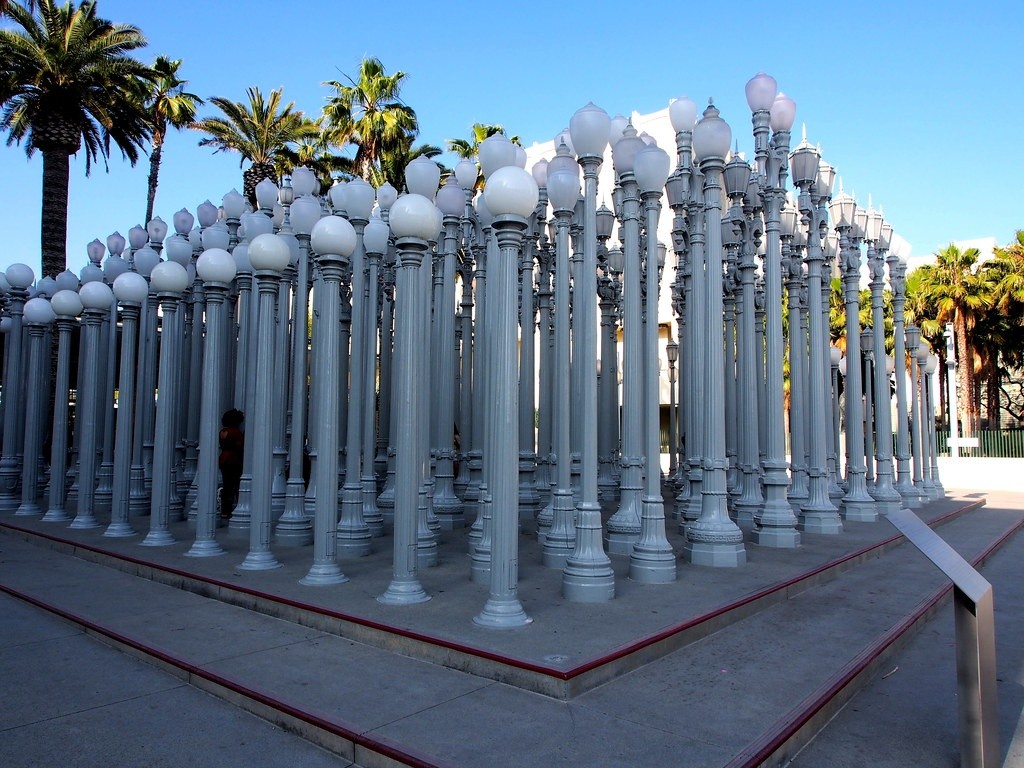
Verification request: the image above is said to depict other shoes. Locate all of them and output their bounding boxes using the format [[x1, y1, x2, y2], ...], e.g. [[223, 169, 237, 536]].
[[220, 509, 234, 518]]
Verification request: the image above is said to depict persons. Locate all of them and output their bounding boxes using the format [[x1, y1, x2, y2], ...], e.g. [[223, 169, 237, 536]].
[[217, 409, 245, 520]]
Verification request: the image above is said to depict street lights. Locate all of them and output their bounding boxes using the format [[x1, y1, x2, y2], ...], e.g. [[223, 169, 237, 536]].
[[376, 193, 438, 608], [471, 166, 531, 629], [183, 248, 237, 559], [297, 216, 351, 586], [138, 260, 189, 547], [67, 282, 113, 529], [238, 234, 292, 572], [10, 71, 944, 581], [0, 263, 35, 512], [561, 102, 616, 600], [101, 271, 149, 537], [12, 290, 83, 521]]
